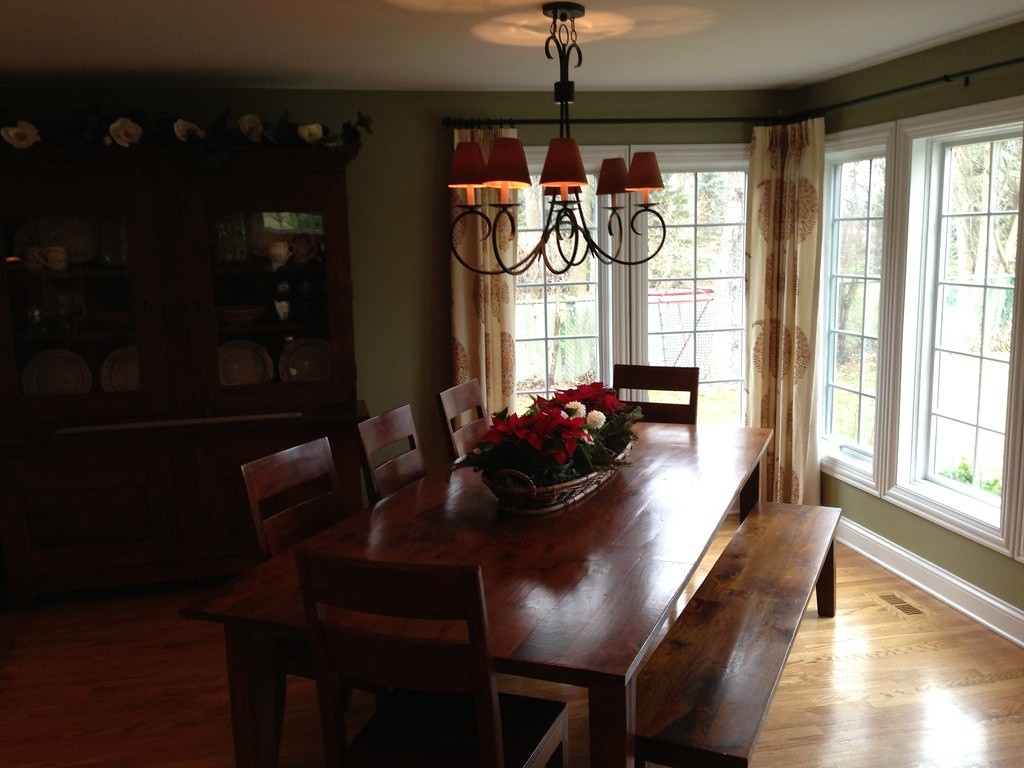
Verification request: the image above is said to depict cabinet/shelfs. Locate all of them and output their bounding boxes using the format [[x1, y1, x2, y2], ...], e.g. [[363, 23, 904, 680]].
[[0, 142, 357, 613]]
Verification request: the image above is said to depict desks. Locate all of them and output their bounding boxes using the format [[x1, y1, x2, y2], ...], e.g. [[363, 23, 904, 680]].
[[175, 420, 773, 768]]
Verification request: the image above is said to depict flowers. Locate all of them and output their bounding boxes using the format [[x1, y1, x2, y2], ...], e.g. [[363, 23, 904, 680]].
[[0, 105, 375, 170], [453, 374, 644, 488]]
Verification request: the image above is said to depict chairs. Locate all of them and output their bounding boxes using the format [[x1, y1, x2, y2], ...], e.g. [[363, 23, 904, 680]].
[[296, 545, 568, 768], [354, 403, 427, 506], [230, 436, 380, 768], [437, 377, 489, 462]]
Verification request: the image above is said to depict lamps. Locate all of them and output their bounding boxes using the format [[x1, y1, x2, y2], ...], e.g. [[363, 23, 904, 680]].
[[448, 2, 666, 275]]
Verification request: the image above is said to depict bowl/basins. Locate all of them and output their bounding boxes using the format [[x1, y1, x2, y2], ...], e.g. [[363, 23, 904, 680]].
[[216, 306, 266, 324]]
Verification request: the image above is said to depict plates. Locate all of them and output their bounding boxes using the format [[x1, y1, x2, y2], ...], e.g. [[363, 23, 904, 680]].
[[218, 340, 273, 386], [101, 345, 138, 392], [22, 350, 92, 400], [14, 222, 40, 261], [279, 337, 331, 384]]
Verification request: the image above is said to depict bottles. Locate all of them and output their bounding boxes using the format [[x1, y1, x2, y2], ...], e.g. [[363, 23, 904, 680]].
[[43, 233, 67, 269]]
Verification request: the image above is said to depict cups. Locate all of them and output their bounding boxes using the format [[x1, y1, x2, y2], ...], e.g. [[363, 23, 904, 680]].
[[265, 239, 317, 266]]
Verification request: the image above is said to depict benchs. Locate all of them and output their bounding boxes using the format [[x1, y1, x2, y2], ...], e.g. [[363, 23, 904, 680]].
[[634, 500, 843, 768]]
